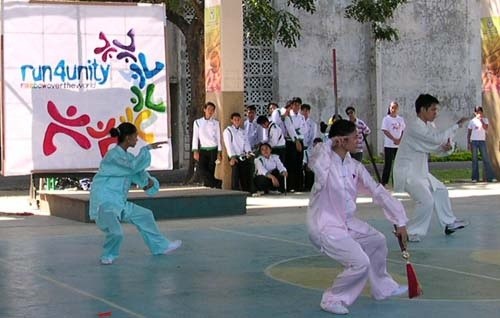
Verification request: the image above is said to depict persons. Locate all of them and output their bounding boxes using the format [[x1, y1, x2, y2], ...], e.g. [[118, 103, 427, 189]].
[[191, 97, 372, 198], [89, 122, 182, 265], [307, 120, 410, 315], [393, 93, 473, 241], [468, 106, 497, 183], [381, 102, 407, 186]]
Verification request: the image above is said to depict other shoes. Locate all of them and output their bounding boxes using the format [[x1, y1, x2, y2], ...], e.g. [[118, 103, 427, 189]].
[[101, 257, 112, 264], [163, 240, 182, 254], [444, 219, 469, 235], [320, 301, 349, 314], [488, 178, 497, 181], [252, 191, 264, 197], [269, 190, 281, 195], [472, 179, 477, 182], [391, 287, 408, 295], [409, 235, 421, 242]]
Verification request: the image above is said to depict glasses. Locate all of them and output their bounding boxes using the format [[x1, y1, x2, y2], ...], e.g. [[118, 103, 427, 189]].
[[346, 111, 353, 115]]
